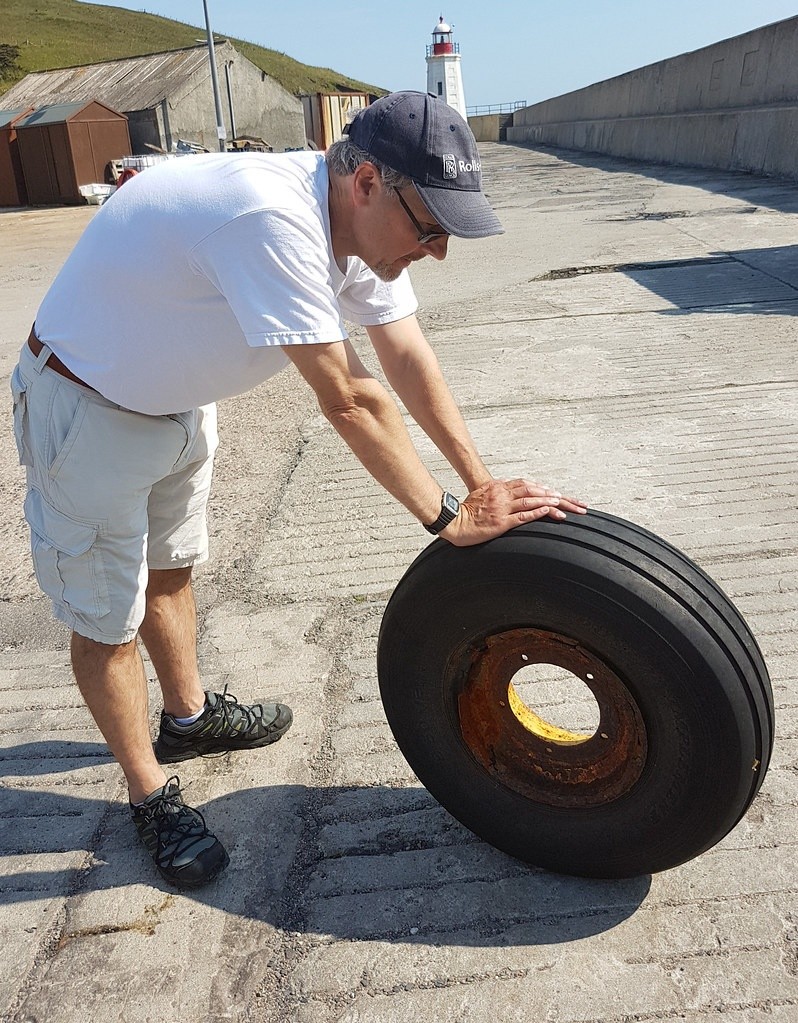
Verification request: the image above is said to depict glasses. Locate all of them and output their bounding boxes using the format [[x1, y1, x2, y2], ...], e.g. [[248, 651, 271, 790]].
[[393, 184, 452, 245]]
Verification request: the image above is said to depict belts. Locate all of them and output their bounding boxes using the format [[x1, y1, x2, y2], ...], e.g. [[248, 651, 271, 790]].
[[28, 320, 94, 389]]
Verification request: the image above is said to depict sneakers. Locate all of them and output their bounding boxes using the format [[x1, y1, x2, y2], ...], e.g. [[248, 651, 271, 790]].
[[156, 683, 294, 764], [128, 775, 231, 888]]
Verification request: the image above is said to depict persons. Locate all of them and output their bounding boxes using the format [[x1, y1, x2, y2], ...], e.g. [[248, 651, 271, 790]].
[[9, 90, 590, 889]]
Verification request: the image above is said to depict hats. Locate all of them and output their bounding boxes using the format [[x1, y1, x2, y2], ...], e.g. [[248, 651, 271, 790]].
[[342, 89, 506, 239]]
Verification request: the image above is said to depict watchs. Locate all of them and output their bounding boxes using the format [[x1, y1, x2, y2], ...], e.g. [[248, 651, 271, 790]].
[[422, 491, 460, 535]]
